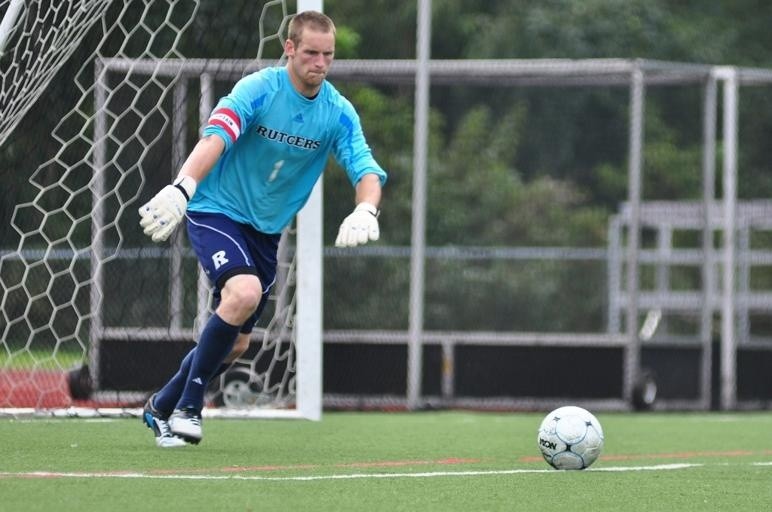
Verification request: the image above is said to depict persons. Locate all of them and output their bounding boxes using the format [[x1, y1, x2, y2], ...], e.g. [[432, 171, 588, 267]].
[[139, 11, 389, 448]]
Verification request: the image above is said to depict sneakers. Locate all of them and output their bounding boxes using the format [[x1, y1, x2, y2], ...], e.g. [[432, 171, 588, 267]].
[[166, 408, 204, 445], [142, 393, 189, 448]]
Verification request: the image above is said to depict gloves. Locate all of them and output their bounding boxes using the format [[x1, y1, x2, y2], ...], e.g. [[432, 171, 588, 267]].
[[334, 202, 381, 248], [138, 175, 197, 244]]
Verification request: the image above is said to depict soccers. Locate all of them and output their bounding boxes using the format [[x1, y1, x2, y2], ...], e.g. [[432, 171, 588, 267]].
[[538, 406, 604, 471]]
